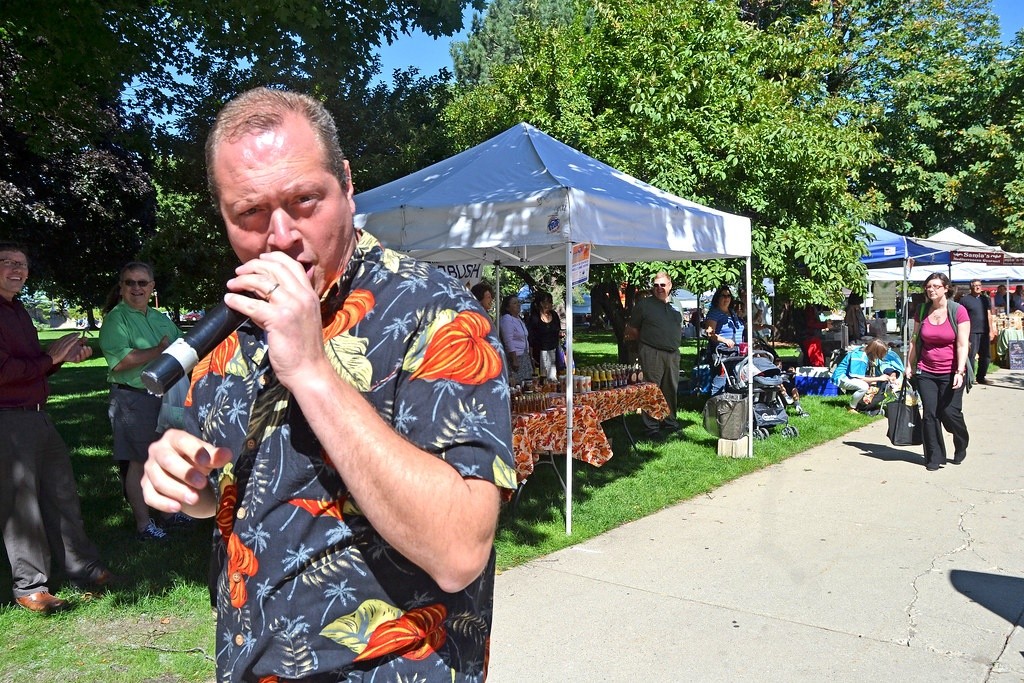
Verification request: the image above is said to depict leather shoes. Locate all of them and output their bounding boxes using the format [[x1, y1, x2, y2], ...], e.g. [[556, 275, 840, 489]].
[[16, 590, 69, 614], [95, 568, 131, 591]]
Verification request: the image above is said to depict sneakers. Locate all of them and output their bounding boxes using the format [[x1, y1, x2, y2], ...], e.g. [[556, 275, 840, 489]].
[[158, 511, 204, 529], [136, 518, 175, 544]]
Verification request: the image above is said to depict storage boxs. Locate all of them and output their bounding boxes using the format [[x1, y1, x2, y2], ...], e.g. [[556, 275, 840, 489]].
[[795, 366, 839, 397], [1006, 340, 1024, 370]]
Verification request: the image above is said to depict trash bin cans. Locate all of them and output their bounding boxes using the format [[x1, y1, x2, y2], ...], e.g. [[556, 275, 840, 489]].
[[718, 393, 749, 440]]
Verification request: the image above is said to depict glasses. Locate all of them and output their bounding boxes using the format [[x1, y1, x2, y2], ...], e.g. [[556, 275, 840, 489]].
[[0, 259, 28, 269], [927, 285, 944, 289], [654, 284, 666, 287], [722, 294, 731, 299], [123, 279, 152, 287]]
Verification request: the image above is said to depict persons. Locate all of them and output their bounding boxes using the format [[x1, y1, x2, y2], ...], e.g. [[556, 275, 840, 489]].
[[774, 357, 803, 413], [996, 285, 1024, 313], [802, 302, 832, 367], [903, 272, 969, 470], [501, 296, 533, 385], [526, 293, 561, 381], [958, 279, 993, 385], [706, 285, 745, 396], [471, 284, 493, 311], [844, 289, 866, 340], [830, 338, 900, 414], [631, 273, 684, 439], [99, 265, 194, 542], [140, 87, 519, 683], [0, 243, 121, 612]]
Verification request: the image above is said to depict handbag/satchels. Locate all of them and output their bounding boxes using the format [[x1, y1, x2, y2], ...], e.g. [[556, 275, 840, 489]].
[[557, 341, 576, 375], [508, 350, 533, 385], [887, 376, 922, 446]]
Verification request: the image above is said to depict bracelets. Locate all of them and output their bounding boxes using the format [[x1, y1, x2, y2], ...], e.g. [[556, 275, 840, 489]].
[[955, 370, 965, 376]]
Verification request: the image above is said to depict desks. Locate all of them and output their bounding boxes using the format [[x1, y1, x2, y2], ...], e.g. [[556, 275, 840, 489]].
[[500, 382, 670, 517]]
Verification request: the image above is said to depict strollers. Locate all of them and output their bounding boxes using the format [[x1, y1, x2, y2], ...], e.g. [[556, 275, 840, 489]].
[[702, 343, 798, 440]]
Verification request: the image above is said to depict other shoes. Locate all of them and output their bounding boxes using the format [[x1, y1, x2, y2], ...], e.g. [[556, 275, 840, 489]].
[[976, 378, 994, 385], [785, 395, 794, 405], [651, 432, 664, 441], [926, 459, 946, 471], [954, 449, 966, 464]]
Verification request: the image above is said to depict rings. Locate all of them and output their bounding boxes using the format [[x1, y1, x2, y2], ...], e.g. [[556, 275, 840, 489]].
[[266, 283, 279, 300]]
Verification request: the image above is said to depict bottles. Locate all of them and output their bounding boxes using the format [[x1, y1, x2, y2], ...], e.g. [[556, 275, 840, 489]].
[[509, 362, 644, 416]]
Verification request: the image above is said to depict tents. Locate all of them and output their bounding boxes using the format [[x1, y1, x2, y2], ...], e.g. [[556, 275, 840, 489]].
[[514, 222, 952, 371], [352, 123, 754, 536], [865, 228, 1024, 334]]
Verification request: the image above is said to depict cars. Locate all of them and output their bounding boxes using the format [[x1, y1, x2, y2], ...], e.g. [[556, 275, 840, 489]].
[[670, 287, 733, 327], [518, 285, 592, 325], [184, 311, 202, 322]]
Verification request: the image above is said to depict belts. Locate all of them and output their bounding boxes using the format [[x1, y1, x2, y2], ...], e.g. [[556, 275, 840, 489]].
[[0, 403, 43, 412], [117, 383, 130, 391]]
